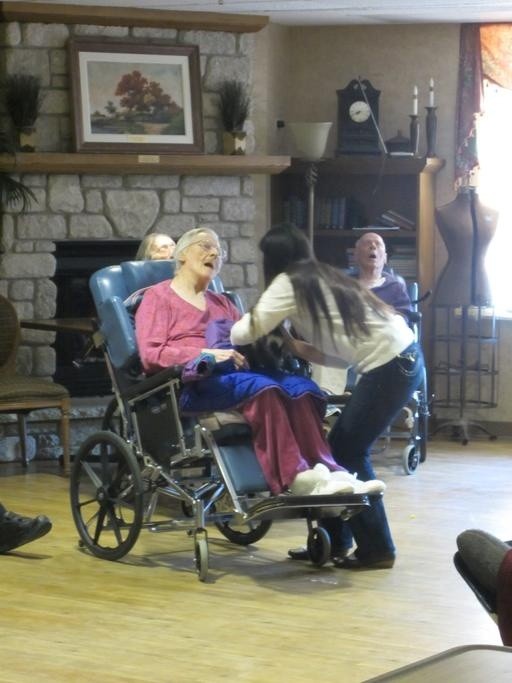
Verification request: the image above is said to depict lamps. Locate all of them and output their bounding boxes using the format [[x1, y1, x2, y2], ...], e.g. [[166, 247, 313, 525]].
[[288, 120, 333, 248]]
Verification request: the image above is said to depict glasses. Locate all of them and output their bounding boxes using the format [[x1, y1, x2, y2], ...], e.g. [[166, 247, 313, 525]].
[[177, 239, 229, 259]]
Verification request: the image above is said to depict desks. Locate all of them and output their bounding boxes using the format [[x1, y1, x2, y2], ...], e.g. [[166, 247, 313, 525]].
[[19, 315, 214, 476]]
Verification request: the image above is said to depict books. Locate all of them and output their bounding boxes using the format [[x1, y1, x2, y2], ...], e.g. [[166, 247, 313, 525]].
[[278, 185, 420, 282]]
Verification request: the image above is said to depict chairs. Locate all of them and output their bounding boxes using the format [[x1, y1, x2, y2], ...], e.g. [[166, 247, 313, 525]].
[[0, 294, 71, 478]]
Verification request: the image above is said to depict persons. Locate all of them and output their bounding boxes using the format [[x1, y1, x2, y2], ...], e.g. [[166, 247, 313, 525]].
[[135, 223, 388, 501], [312, 227, 419, 435], [229, 226, 427, 570], [490, 538, 512, 650], [129, 230, 177, 262], [432, 184, 501, 317], [0, 502, 57, 557]]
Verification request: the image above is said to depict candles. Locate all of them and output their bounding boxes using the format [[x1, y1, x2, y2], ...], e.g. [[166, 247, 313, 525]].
[[411, 84, 418, 114], [428, 76, 434, 106]]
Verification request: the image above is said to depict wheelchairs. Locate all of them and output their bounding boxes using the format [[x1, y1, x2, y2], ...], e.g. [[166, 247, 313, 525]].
[[325, 270, 434, 473], [70, 259, 383, 581]]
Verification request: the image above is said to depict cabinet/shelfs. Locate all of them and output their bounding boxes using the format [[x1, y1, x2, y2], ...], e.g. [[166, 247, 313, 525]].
[[269, 154, 444, 440]]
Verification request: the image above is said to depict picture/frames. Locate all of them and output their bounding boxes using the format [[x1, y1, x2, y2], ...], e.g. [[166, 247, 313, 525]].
[[67, 39, 207, 155]]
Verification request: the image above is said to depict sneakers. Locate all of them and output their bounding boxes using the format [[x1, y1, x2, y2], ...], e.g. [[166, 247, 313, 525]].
[[0, 510, 52, 553]]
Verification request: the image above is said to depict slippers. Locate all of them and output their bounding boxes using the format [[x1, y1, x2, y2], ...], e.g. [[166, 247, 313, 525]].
[[289, 462, 387, 495]]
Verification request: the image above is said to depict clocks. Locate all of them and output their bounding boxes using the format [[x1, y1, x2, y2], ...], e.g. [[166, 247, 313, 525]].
[[334, 78, 383, 156]]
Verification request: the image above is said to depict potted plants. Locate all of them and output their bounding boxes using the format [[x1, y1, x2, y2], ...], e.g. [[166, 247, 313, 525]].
[[3, 70, 48, 151], [216, 75, 252, 154]]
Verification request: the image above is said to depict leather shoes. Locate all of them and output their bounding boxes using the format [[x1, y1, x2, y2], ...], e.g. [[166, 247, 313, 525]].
[[288, 543, 348, 560], [332, 552, 395, 569]]
